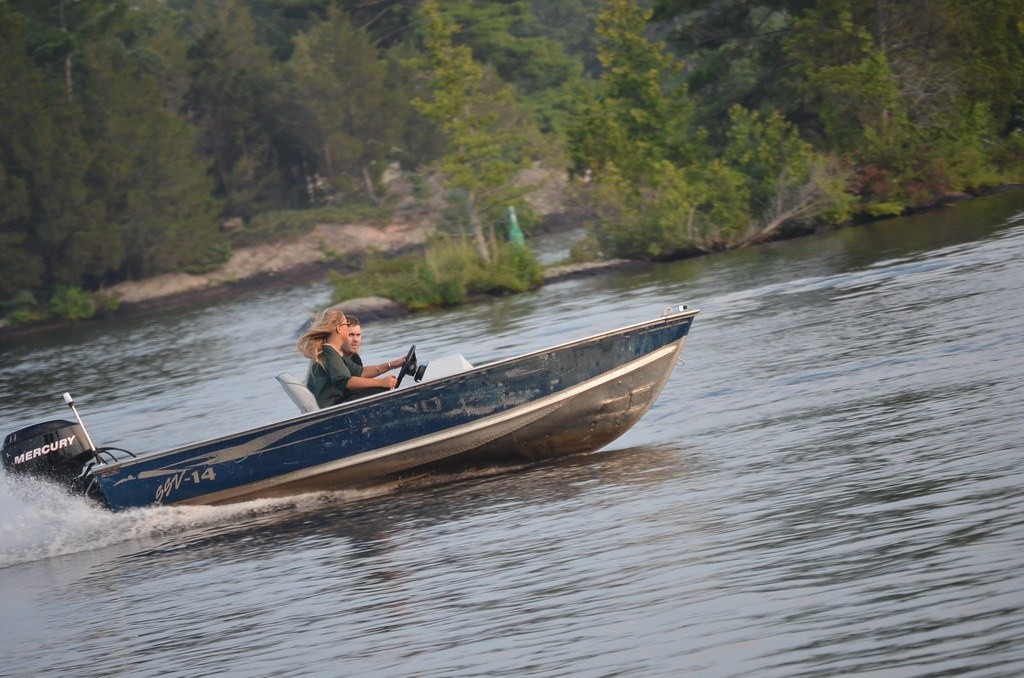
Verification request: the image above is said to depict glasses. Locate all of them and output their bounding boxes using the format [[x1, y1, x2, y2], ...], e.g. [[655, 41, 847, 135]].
[[339, 320, 351, 328]]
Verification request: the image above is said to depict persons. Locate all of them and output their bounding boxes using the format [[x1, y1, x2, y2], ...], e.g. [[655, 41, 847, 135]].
[[340, 315, 363, 366], [297, 309, 407, 409]]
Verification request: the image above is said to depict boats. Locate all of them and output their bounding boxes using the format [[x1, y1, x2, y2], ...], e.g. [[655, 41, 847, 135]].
[[1, 304, 701, 516]]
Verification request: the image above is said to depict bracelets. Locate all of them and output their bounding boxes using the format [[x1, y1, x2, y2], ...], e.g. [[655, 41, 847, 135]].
[[388, 362, 391, 370], [377, 366, 379, 374]]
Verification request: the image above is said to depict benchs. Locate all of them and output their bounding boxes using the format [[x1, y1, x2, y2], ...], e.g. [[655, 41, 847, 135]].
[[276, 372, 324, 414]]
